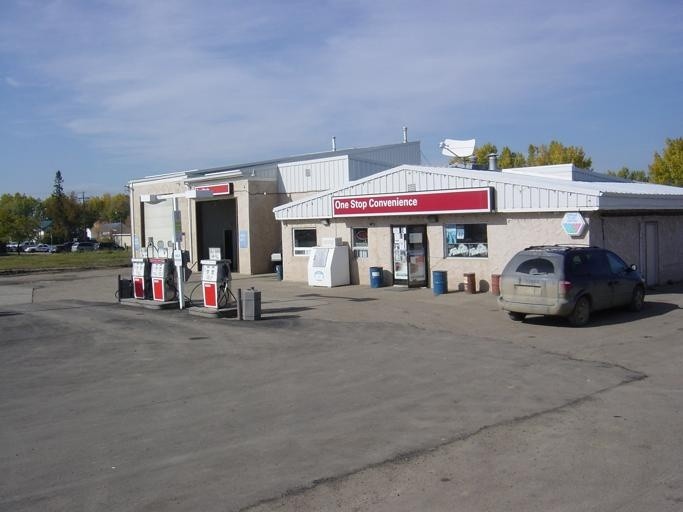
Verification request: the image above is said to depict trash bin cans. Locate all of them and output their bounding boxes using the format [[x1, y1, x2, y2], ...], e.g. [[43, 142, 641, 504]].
[[276, 265, 283, 281]]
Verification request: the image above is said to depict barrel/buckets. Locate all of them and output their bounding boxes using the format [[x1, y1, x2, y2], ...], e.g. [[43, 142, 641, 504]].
[[491, 274, 500, 296], [458, 283, 464, 290], [277, 265, 281, 280]]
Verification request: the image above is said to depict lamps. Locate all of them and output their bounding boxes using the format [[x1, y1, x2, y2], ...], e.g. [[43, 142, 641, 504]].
[[427, 215, 437, 223], [320, 219, 328, 225]]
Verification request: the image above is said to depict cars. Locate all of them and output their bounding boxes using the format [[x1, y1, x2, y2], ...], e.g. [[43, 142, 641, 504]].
[[7, 239, 125, 253], [499, 243, 647, 326]]
